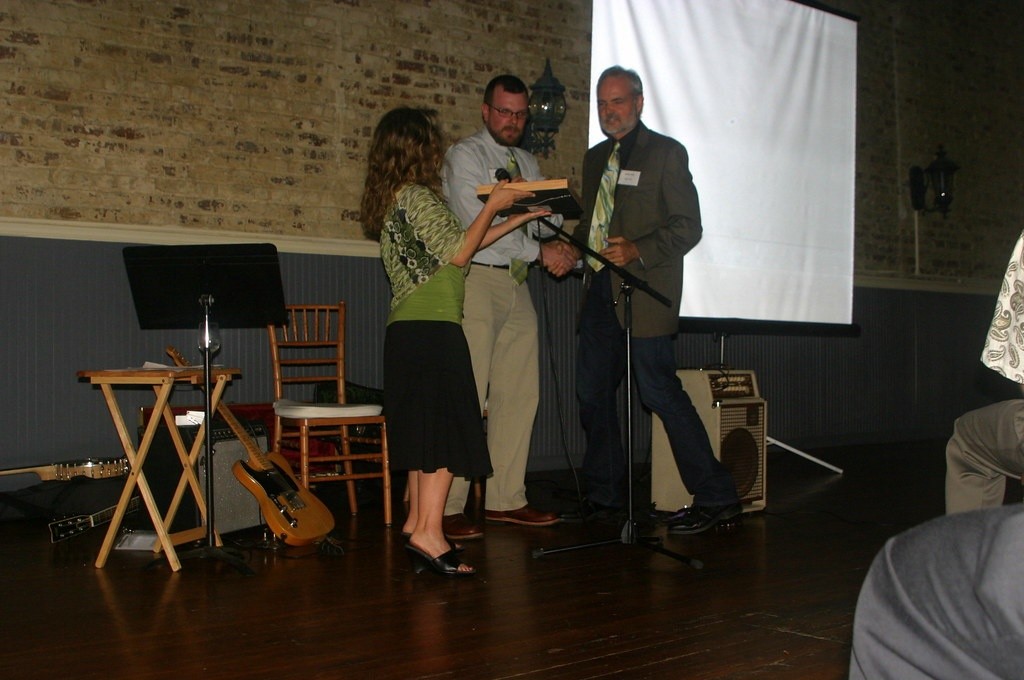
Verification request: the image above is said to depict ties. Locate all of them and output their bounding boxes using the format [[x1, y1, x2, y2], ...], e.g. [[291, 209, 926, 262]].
[[505, 150, 527, 287], [586, 142, 620, 272]]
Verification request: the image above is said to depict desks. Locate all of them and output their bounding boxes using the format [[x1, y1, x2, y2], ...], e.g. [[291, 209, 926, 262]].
[[77, 367, 241, 572]]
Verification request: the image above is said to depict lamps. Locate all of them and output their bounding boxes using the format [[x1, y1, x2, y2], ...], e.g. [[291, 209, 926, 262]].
[[909, 144, 961, 218], [515, 58, 568, 159]]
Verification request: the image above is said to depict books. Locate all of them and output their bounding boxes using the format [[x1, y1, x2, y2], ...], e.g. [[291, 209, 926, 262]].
[[477, 178, 585, 220]]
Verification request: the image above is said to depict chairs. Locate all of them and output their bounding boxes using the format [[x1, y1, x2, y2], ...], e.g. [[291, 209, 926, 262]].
[[266, 299, 393, 528]]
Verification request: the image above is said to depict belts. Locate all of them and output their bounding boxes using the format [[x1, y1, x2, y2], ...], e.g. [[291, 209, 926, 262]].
[[471, 261, 509, 269]]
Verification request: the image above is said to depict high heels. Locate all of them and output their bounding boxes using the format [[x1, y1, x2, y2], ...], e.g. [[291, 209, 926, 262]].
[[401, 530, 476, 576]]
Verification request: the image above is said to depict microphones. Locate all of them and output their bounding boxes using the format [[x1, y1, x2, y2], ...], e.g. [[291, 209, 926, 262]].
[[492, 167, 529, 207]]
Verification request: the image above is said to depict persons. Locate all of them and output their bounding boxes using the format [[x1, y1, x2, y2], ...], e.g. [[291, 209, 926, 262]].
[[943, 226, 1024, 513], [544, 65, 742, 536], [438, 73, 576, 541], [359, 107, 551, 577], [850, 500, 1024, 680]]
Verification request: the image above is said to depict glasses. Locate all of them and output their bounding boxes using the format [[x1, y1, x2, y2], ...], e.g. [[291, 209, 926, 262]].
[[487, 103, 528, 119]]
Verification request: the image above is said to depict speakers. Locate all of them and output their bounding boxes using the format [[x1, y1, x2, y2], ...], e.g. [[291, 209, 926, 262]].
[[136, 419, 272, 535], [652, 369, 767, 512]]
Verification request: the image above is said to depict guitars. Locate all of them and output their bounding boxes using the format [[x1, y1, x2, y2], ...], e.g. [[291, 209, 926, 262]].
[[0, 455, 130, 484], [46, 495, 145, 547], [162, 344, 337, 547]]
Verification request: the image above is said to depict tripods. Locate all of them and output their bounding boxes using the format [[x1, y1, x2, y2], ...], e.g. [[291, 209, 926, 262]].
[[123, 241, 289, 576], [518, 203, 703, 571]]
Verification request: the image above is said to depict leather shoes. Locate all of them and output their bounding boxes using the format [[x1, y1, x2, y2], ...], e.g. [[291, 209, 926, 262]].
[[484, 505, 560, 527], [442, 513, 484, 540], [665, 498, 742, 536], [560, 499, 624, 525]]
